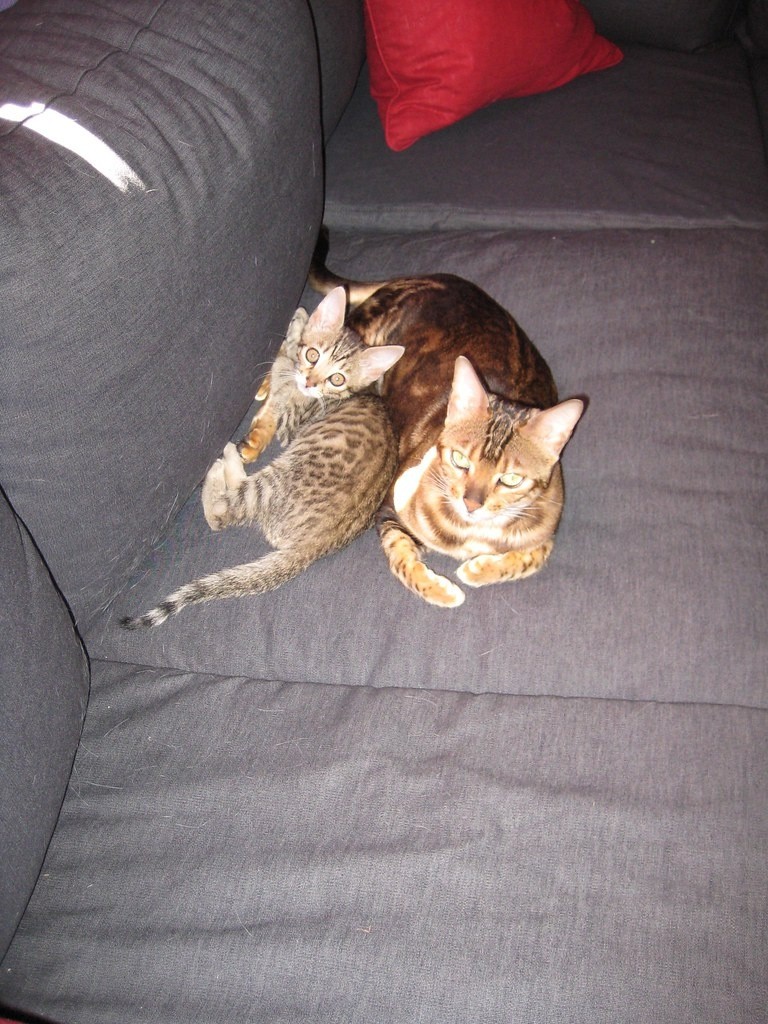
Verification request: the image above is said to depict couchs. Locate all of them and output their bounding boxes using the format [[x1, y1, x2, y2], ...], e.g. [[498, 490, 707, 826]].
[[0, 0, 768, 1024]]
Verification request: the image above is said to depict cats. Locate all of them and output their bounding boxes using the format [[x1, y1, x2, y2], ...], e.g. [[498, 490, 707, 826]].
[[308, 223, 586, 610], [112, 287, 405, 630]]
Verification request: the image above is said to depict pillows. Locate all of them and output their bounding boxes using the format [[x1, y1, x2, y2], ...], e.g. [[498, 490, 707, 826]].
[[364, 0, 624, 154]]
